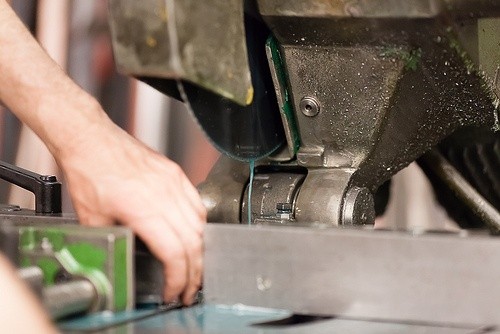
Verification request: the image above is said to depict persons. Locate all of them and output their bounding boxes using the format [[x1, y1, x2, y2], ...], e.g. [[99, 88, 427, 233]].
[[2, 0, 208, 333]]
